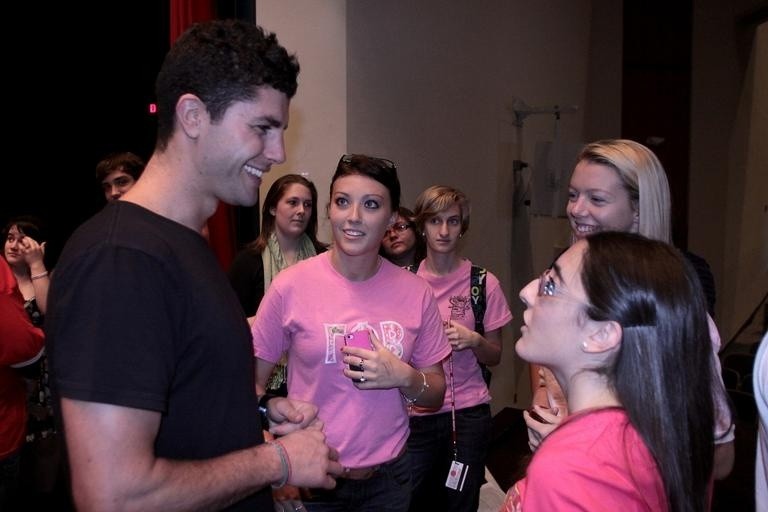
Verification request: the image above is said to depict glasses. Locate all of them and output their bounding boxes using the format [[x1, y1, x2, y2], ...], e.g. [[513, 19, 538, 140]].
[[338, 154, 395, 170], [383, 224, 411, 232], [537, 269, 595, 309]]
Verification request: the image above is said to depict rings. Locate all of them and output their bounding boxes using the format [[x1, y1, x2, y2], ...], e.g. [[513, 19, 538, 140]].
[[23, 243, 30, 249], [359, 358, 366, 371], [359, 372, 365, 381]]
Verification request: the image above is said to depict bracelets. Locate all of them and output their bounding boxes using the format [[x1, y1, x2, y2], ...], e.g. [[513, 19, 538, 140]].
[[29, 269, 49, 280], [262, 437, 295, 491], [257, 392, 281, 432], [400, 369, 428, 405]]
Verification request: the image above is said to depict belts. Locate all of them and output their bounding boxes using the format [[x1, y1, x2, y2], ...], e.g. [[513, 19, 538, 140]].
[[334, 442, 408, 480]]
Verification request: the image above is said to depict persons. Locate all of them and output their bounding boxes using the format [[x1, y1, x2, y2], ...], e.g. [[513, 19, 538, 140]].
[[500, 227, 716, 512], [5, 219, 56, 451], [403, 183, 514, 510], [751, 330, 768, 511], [1, 251, 47, 511], [93, 153, 146, 205], [374, 207, 429, 277], [228, 172, 332, 318], [248, 154, 452, 511], [42, 15, 347, 511], [522, 139, 736, 480]]
